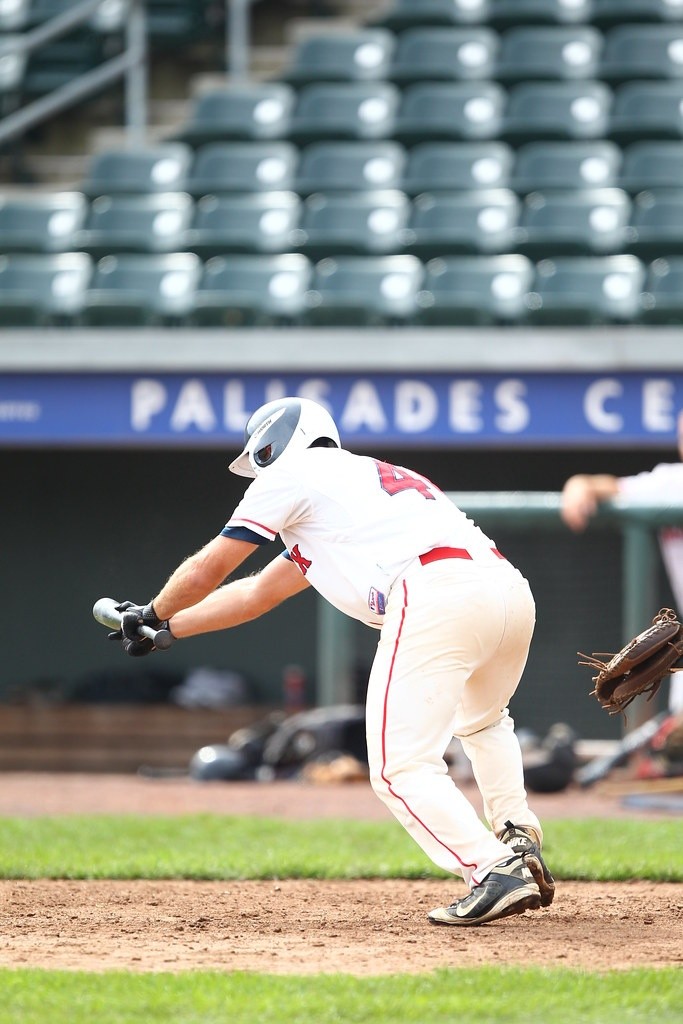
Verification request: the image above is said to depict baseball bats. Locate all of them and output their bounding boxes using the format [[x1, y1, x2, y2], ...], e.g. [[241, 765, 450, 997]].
[[95, 598, 172, 650]]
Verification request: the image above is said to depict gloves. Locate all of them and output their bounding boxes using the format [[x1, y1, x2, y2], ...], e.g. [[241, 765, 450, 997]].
[[120, 618, 177, 657], [106, 601, 165, 642]]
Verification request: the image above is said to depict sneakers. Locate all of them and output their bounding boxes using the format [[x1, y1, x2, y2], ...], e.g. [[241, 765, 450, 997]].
[[499, 821, 555, 907], [427, 847, 541, 925]]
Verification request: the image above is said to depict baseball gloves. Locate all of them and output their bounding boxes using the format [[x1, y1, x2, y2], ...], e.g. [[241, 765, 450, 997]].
[[578, 606, 682, 730]]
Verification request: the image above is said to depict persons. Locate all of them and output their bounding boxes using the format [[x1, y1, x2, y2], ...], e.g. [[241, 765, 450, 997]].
[[107, 397, 554, 924], [560, 461, 683, 532]]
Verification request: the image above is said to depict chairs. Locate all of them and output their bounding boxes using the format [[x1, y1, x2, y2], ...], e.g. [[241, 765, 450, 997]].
[[0, 0, 682, 327]]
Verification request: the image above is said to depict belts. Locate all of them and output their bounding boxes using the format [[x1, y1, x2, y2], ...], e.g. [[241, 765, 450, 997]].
[[419, 547, 504, 566]]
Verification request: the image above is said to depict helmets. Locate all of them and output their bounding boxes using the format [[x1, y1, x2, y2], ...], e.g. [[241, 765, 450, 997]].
[[228, 397, 340, 479]]
